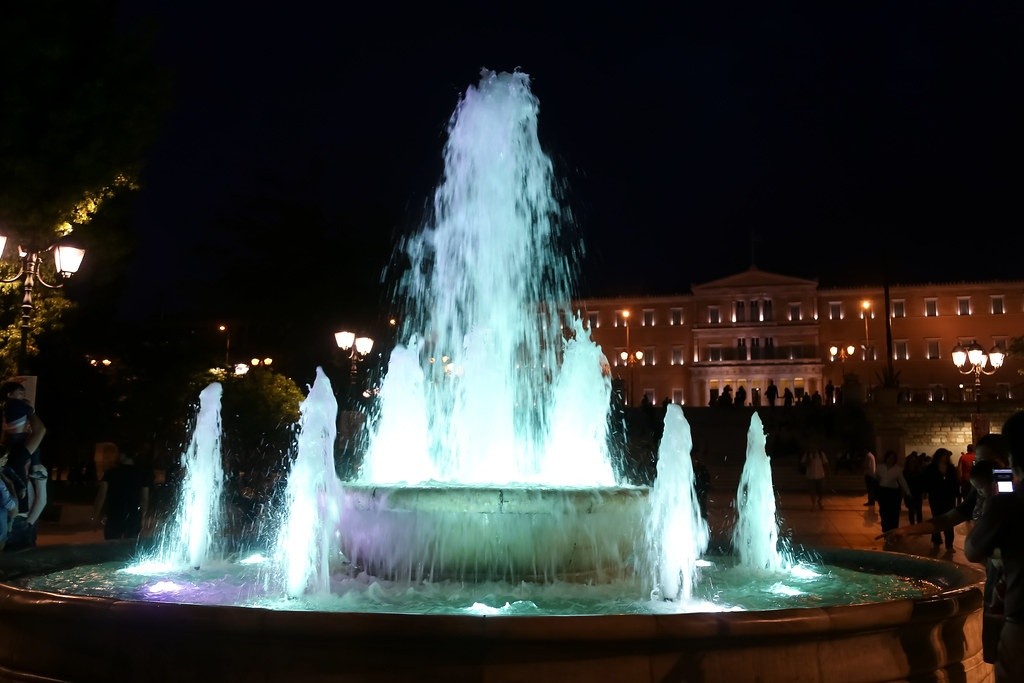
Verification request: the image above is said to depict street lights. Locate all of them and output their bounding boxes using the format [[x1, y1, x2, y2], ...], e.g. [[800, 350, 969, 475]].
[[861, 299, 874, 402], [219, 324, 231, 363], [829, 344, 857, 378], [951, 339, 1007, 446], [0, 223, 89, 379], [333, 327, 377, 400], [622, 309, 632, 409], [621, 350, 645, 409]]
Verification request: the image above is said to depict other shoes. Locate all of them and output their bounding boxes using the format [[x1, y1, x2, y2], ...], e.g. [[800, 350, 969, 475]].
[[933, 543, 940, 552], [948, 548, 956, 553], [29, 471, 46, 479]]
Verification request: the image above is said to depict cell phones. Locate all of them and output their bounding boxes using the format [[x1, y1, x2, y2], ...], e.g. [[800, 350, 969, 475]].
[[991, 468, 1014, 495]]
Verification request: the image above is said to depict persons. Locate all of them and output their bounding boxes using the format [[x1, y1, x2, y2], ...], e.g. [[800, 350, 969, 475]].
[[0, 381, 272, 557], [642, 379, 1024, 683]]
